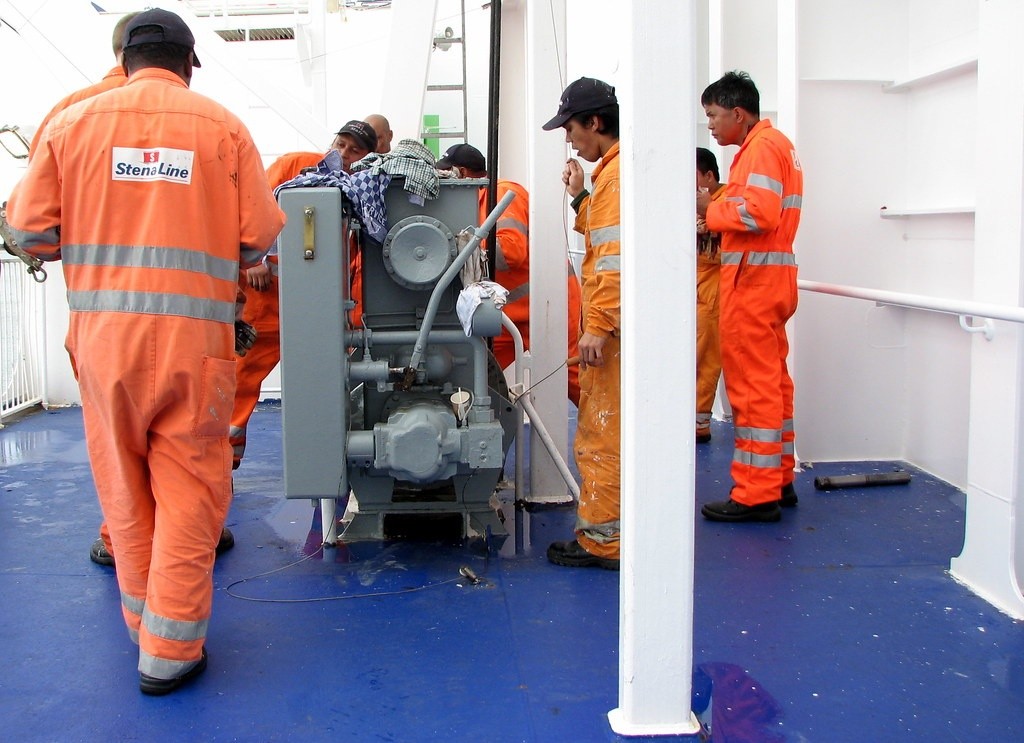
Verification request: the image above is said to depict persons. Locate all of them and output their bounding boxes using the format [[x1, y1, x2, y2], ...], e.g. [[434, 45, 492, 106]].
[[434, 77, 621, 571], [5, 7, 395, 697], [696, 68, 804, 524]]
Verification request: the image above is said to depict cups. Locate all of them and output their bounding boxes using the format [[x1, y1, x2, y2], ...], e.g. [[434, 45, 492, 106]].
[[450, 391, 470, 420]]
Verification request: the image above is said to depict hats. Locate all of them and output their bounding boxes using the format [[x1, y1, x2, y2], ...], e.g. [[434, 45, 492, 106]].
[[123, 7, 201, 68], [542, 76, 617, 130], [335, 120, 377, 152], [435, 143, 485, 172]]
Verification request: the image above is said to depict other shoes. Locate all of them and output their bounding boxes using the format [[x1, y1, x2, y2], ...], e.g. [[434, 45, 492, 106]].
[[215, 527, 234, 552], [702, 481, 798, 523], [140, 647, 207, 695], [547, 539, 620, 571], [89, 538, 115, 566], [696, 434, 711, 442]]
